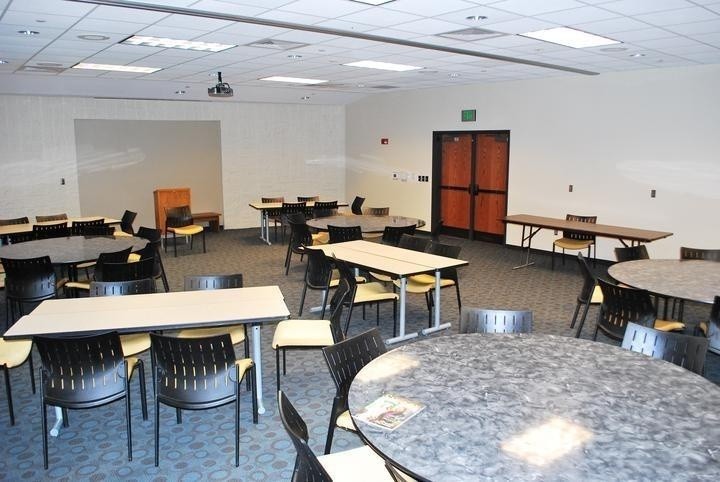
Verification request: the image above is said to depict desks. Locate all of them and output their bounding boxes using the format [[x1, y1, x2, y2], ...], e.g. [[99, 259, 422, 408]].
[[608, 259, 720, 333], [348, 334, 720, 482], [497, 214, 672, 270]]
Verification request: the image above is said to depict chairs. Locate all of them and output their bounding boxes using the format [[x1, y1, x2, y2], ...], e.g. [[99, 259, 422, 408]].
[[569, 252, 616, 338], [672, 247, 720, 322], [459, 307, 532, 334], [593, 277, 686, 342], [694, 295, 720, 376], [149, 331, 258, 467], [620, 321, 710, 377], [276, 390, 396, 482], [614, 245, 672, 321], [32, 329, 147, 470], [552, 214, 597, 270], [384, 459, 423, 482], [321, 328, 387, 455]]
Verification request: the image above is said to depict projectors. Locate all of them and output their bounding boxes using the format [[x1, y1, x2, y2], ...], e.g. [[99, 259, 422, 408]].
[[207, 83, 234, 98]]
